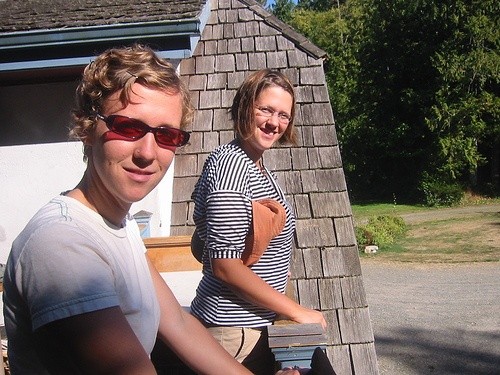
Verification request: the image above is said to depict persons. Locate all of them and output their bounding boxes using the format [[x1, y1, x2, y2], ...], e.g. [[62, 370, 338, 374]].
[[2, 43, 310, 375], [190, 69, 328, 375]]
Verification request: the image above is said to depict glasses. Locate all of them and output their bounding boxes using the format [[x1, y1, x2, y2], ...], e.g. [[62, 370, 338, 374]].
[[92, 108, 190, 146], [254, 103, 293, 124]]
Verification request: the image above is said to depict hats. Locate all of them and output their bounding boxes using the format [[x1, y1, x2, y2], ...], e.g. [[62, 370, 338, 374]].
[[190, 198, 287, 268]]
